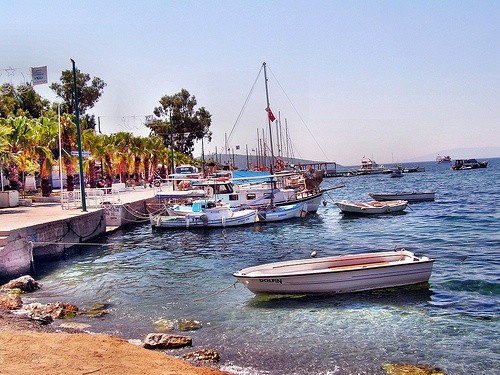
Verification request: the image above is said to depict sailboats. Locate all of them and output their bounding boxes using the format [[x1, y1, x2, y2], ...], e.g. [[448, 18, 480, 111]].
[[149, 61, 325, 227]]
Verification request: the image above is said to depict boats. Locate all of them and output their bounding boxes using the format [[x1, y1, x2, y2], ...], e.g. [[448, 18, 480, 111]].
[[382, 166, 425, 172], [357, 155, 393, 173], [440, 156, 451, 162], [390, 170, 404, 178], [451, 158, 488, 169], [334, 199, 408, 212], [233, 248, 434, 293], [368, 192, 435, 203]]
